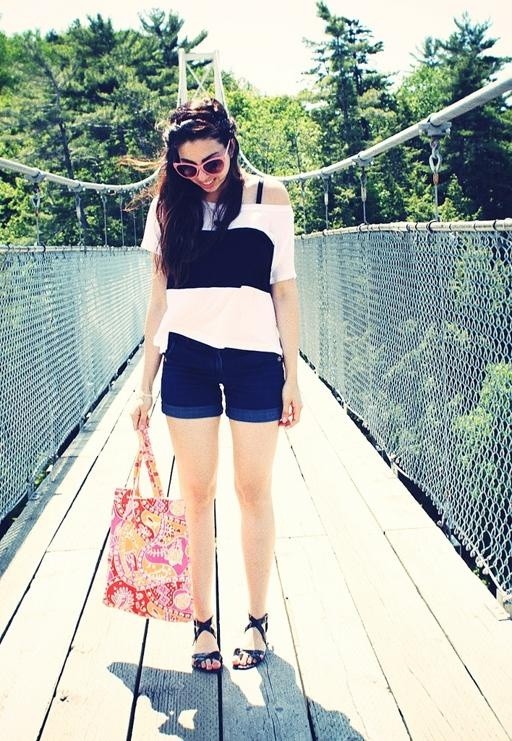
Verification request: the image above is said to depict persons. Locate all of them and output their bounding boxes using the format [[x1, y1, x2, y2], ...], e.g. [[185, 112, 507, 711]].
[[131, 96, 304, 672]]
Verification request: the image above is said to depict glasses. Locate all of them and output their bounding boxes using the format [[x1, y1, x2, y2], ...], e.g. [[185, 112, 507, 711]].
[[172, 140, 232, 179]]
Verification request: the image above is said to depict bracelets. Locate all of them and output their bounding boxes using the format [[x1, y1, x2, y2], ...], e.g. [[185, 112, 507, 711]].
[[137, 390, 153, 400]]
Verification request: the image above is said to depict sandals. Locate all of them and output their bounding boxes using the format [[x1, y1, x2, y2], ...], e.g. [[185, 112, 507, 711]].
[[232, 613, 269, 670], [192, 617, 222, 672]]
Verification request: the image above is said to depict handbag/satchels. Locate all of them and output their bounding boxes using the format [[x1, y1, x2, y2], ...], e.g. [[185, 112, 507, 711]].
[[101, 487, 196, 622]]
